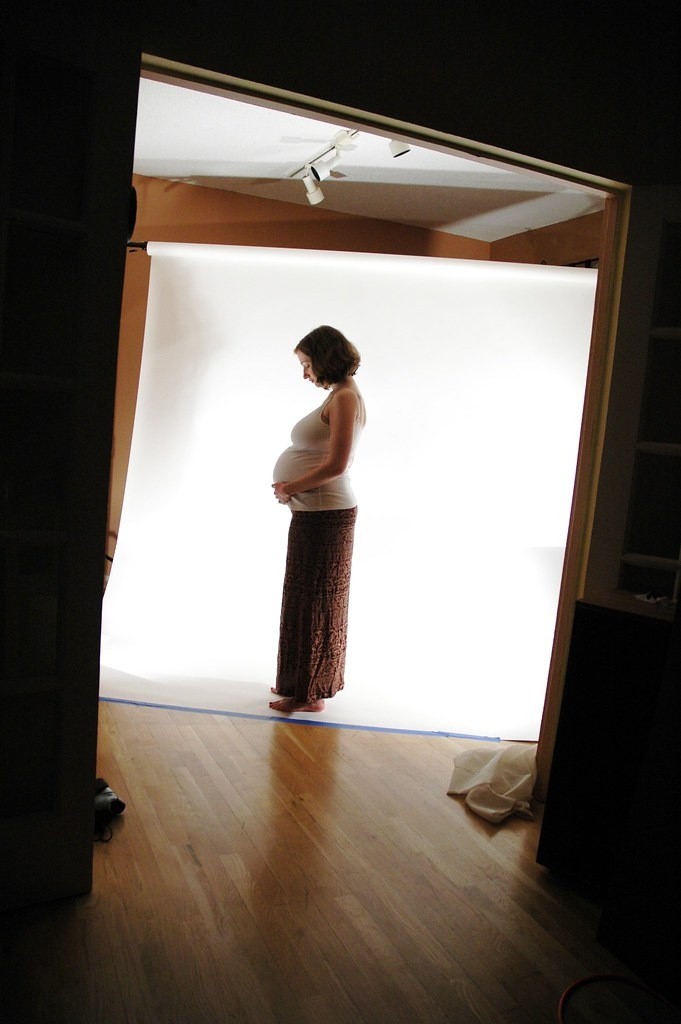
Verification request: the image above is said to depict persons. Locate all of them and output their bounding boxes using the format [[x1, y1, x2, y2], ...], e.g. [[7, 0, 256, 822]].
[[269, 325, 366, 713]]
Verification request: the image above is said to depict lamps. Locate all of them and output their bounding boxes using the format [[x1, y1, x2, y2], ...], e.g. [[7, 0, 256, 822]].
[[311, 135, 357, 183], [389, 139, 411, 158], [303, 165, 324, 205]]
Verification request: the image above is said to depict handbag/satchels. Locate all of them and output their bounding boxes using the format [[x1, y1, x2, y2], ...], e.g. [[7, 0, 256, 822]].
[[96, 778, 126, 843]]
